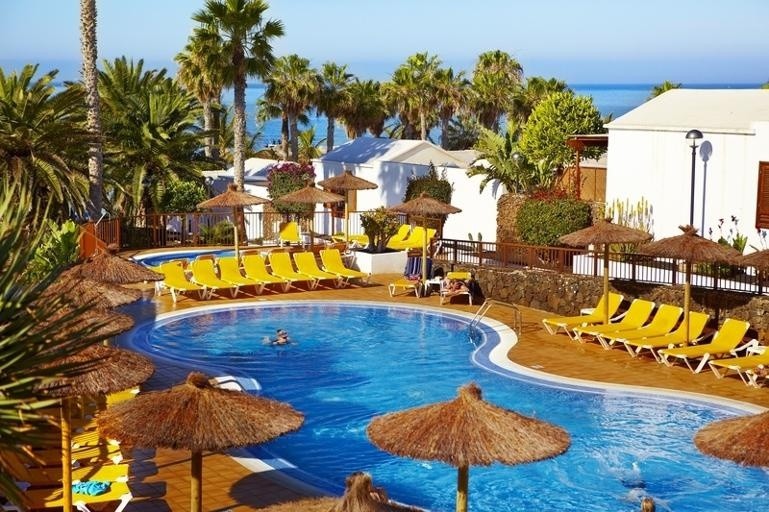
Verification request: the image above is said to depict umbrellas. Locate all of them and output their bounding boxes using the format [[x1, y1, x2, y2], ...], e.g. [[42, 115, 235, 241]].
[[318, 171, 377, 243], [390, 193, 461, 281], [97, 372, 305, 512], [197, 185, 272, 256], [729, 249, 768, 272], [30, 275, 142, 308], [367, 385, 570, 511], [61, 249, 164, 284], [558, 219, 649, 324], [642, 226, 740, 345], [34, 305, 135, 344], [278, 182, 346, 249], [695, 410, 769, 470], [8, 340, 155, 512]]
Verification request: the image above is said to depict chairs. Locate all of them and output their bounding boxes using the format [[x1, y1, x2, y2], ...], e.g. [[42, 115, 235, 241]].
[[542, 292, 623, 340], [0, 383, 140, 512], [708, 346, 769, 386], [746, 365, 769, 389], [658, 318, 758, 374], [580, 308, 594, 316], [624, 311, 710, 364], [600, 305, 683, 357]]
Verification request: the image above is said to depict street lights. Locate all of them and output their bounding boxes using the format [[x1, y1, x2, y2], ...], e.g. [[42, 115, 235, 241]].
[[510, 151, 524, 195], [683, 126, 705, 234]]
